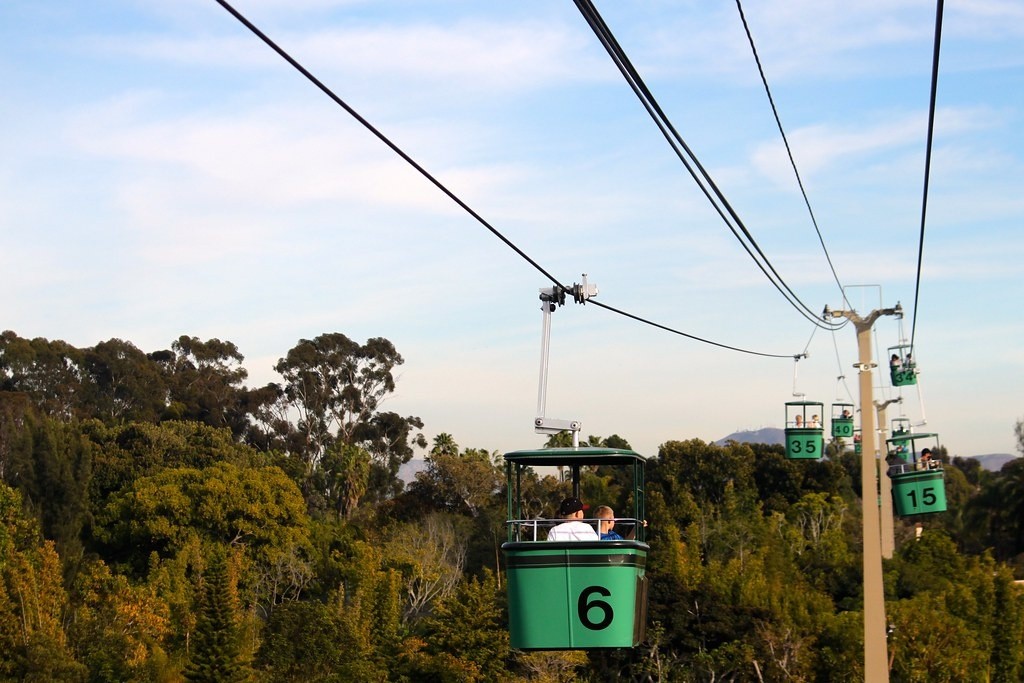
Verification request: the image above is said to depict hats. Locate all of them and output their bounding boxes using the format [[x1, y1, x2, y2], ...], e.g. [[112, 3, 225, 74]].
[[561, 497, 590, 515]]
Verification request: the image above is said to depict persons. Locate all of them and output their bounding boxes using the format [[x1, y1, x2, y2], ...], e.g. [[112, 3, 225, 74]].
[[842, 410, 852, 419], [593, 506, 647, 541], [792, 415, 803, 428], [854, 434, 861, 440], [890, 354, 900, 365], [897, 424, 903, 432], [885, 454, 909, 476], [898, 445, 907, 451], [808, 414, 822, 427], [905, 353, 912, 364], [916, 448, 939, 470], [547, 498, 598, 540]]
